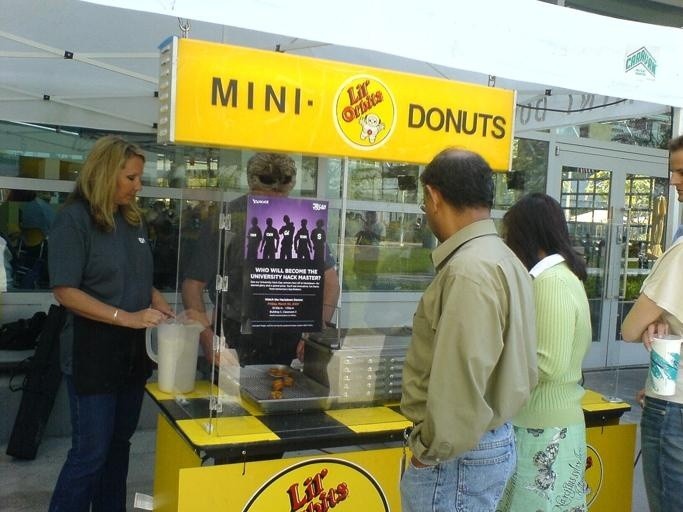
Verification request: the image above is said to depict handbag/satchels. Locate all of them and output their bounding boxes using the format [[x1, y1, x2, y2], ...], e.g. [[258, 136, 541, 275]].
[[0, 311, 46, 351]]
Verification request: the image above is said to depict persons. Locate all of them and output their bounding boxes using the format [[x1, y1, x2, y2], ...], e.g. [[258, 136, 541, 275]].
[[359, 113, 385, 144], [18, 192, 52, 292], [41, 131, 173, 510], [584, 233, 627, 267], [180, 152, 341, 386], [499, 190, 592, 511], [144, 200, 216, 288], [402, 147, 539, 512], [345, 210, 437, 290], [620, 136, 683, 510]]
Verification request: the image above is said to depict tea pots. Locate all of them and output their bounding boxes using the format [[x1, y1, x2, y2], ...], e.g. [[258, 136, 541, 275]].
[[143, 316, 207, 395]]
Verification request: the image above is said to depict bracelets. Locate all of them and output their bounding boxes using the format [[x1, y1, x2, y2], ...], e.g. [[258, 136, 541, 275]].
[[113, 307, 119, 325]]
